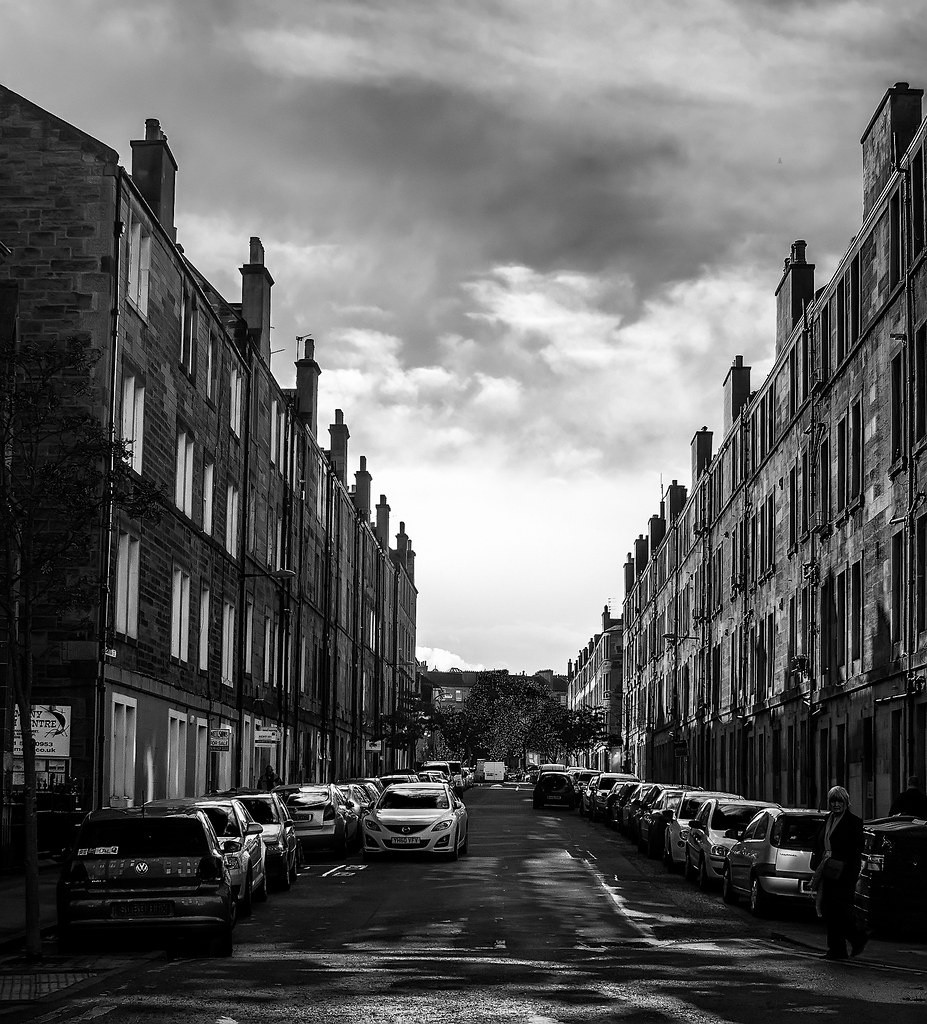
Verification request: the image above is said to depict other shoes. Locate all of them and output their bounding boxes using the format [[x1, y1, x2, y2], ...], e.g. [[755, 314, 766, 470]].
[[848, 933, 871, 956], [819, 949, 850, 959]]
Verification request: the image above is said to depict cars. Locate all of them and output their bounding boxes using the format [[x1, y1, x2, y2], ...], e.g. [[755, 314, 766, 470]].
[[226, 792, 300, 888], [362, 781, 469, 858], [271, 760, 464, 856], [723, 806, 831, 914], [144, 798, 268, 916], [665, 790, 745, 867], [686, 798, 783, 893], [54, 804, 234, 957], [533, 772, 582, 810], [570, 765, 703, 858]]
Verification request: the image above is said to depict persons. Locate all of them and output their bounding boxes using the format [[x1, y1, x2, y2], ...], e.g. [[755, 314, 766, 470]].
[[810, 786, 871, 957], [888, 777, 927, 821]]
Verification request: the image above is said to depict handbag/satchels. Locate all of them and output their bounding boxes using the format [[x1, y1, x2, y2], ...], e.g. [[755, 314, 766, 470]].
[[814, 857, 844, 881]]
